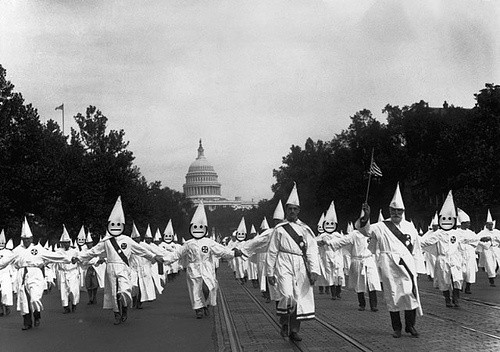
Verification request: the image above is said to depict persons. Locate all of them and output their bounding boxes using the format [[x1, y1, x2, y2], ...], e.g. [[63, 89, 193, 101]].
[[56, 224, 82, 313], [355, 182, 426, 337], [315, 201, 500, 300], [129, 221, 164, 310], [82, 196, 164, 325], [159, 200, 240, 319], [145, 199, 287, 309], [81, 232, 105, 304], [6, 225, 87, 298], [0, 216, 72, 330], [326, 210, 382, 312], [265, 180, 321, 341], [0, 230, 14, 316], [421, 190, 489, 308]]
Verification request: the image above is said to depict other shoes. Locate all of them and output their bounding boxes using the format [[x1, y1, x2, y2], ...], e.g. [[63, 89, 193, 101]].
[[332, 294, 342, 301], [393, 324, 420, 338], [63, 305, 77, 314], [281, 324, 302, 341], [88, 298, 98, 305], [358, 306, 365, 311], [489, 284, 497, 287], [266, 298, 271, 303], [22, 318, 40, 331], [370, 306, 379, 312], [465, 290, 473, 294], [113, 313, 128, 325], [196, 307, 209, 319], [0, 306, 11, 316], [445, 302, 462, 307]]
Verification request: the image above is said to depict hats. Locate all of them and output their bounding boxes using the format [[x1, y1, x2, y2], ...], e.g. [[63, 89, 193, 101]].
[[486, 209, 492, 223], [20, 216, 32, 238], [273, 181, 299, 220], [429, 189, 470, 229], [190, 200, 207, 226], [317, 201, 337, 226], [0, 229, 13, 249], [378, 209, 385, 222], [59, 223, 71, 242], [131, 222, 140, 238], [107, 195, 125, 224], [145, 218, 186, 244], [237, 216, 269, 234], [389, 182, 405, 210], [77, 225, 93, 243]]
